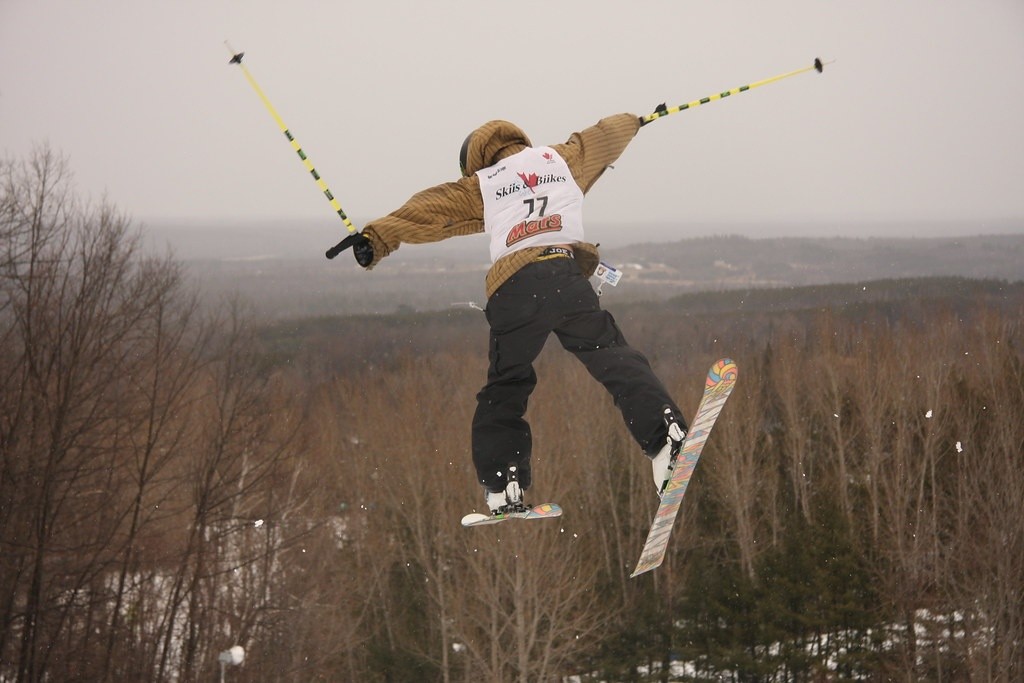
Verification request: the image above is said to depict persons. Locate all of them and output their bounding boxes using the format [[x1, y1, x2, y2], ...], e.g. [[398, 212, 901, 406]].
[[357, 113, 690, 513]]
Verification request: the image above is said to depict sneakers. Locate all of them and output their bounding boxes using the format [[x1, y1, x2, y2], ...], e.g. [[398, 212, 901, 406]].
[[485, 462, 524, 514], [652, 404, 688, 499]]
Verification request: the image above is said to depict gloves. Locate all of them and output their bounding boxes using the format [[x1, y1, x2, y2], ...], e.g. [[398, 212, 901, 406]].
[[326, 233, 374, 267]]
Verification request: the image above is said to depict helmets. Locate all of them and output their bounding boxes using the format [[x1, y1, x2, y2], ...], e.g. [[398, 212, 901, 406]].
[[459, 130, 470, 173]]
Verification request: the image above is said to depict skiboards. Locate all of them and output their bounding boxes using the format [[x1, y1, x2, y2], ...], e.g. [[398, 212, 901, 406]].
[[460, 357, 739, 579]]
